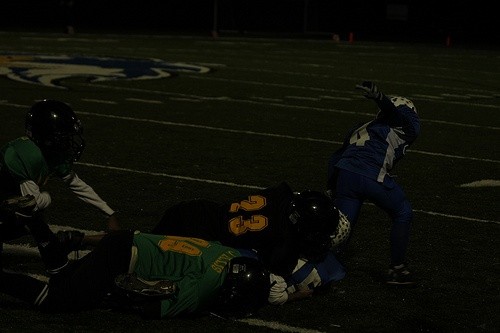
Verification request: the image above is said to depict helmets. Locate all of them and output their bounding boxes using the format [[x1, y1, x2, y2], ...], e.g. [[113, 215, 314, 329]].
[[330, 209, 351, 243], [384, 96, 417, 114], [24, 100, 83, 139], [288, 189, 339, 241]]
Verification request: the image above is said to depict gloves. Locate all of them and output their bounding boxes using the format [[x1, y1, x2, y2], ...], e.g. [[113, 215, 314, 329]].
[[355, 78, 383, 101]]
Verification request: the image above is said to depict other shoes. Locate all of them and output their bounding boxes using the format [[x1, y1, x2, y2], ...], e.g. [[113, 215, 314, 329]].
[[52, 230, 85, 255], [0, 194, 41, 220], [37, 228, 71, 275]]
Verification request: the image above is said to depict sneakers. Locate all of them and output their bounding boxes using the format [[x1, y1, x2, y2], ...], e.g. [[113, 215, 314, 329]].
[[387, 264, 432, 286], [115, 273, 176, 303], [335, 250, 345, 265]]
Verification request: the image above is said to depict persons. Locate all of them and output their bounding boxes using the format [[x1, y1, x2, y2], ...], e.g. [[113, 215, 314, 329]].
[[316, 80, 423, 287], [58, 182, 351, 305], [0, 228, 271, 320], [0, 100, 119, 252]]
